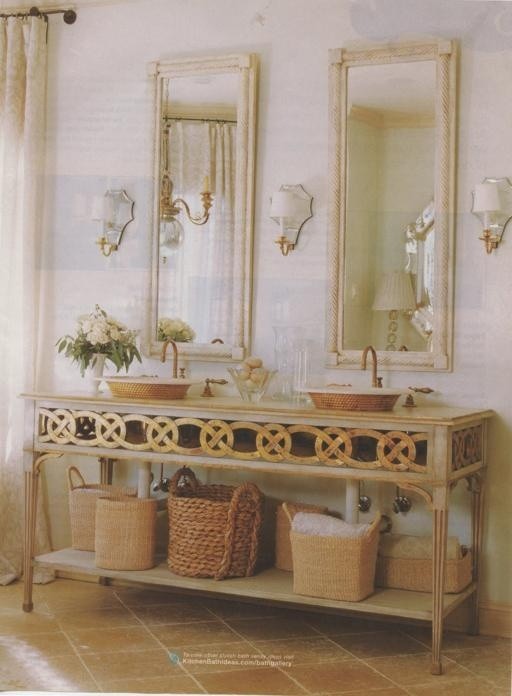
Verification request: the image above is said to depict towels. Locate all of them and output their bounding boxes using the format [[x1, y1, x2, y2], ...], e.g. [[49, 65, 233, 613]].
[[292, 512, 370, 535]]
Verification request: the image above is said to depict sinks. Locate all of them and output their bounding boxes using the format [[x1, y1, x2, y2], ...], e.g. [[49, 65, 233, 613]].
[[305, 387, 402, 413], [102, 374, 192, 402]]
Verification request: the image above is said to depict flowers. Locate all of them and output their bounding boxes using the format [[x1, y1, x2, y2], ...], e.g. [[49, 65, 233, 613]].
[[158, 317, 195, 343], [54, 301, 143, 378]]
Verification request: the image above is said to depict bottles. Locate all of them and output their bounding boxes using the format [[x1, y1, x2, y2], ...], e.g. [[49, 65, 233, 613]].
[[280, 328, 310, 406]]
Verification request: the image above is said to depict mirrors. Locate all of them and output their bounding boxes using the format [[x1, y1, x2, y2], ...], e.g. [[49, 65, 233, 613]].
[[152, 54, 256, 363], [326, 38, 460, 374]]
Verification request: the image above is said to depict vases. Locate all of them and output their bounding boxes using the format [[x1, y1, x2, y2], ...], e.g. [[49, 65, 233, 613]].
[[85, 353, 106, 391]]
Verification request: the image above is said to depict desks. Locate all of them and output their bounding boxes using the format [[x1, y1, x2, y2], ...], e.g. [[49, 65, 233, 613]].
[[19, 394, 496, 677]]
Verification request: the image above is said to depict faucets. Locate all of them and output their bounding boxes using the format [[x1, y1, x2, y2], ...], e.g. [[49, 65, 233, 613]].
[[160, 339, 179, 379], [361, 344, 383, 388], [210, 338, 224, 343], [397, 345, 409, 351]]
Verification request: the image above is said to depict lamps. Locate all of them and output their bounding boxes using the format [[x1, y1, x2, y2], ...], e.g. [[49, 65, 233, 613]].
[[370, 271, 416, 350], [90, 189, 135, 257], [471, 176, 512, 254], [268, 185, 313, 256], [160, 77, 215, 263]]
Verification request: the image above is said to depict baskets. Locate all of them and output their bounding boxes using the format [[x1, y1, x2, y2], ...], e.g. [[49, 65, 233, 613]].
[[66, 464, 154, 552]]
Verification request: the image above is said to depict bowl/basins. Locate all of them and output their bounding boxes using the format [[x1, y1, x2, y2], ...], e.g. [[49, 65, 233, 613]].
[[228, 366, 278, 405]]
